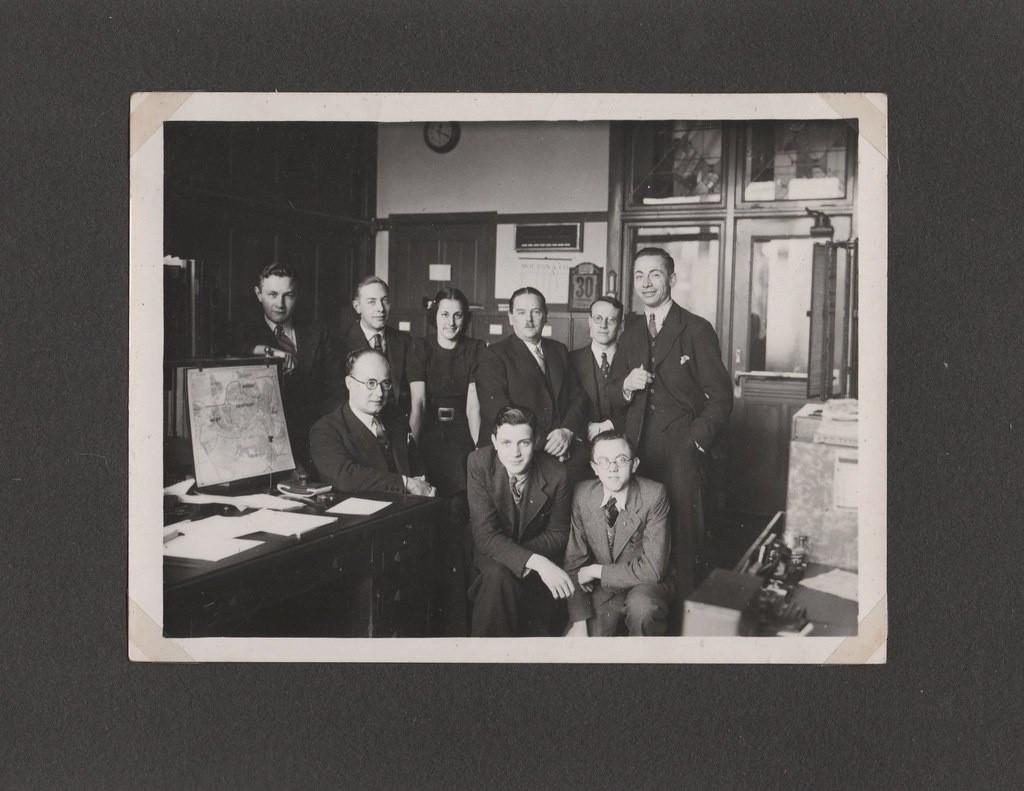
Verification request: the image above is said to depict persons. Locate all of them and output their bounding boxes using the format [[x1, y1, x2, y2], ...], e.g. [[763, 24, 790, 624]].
[[236, 247, 733, 637]]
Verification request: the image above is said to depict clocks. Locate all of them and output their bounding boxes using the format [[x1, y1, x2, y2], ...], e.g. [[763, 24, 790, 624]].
[[423, 122, 460, 152]]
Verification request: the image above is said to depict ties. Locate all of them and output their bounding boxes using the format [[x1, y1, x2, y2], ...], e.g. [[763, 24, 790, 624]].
[[373, 416, 390, 458], [600, 352, 609, 378], [606, 498, 619, 527], [649, 314, 657, 338], [374, 334, 382, 353], [509, 476, 521, 498], [535, 347, 545, 374], [274, 327, 295, 353]]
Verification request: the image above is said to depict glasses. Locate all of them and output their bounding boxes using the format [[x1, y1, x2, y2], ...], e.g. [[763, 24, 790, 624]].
[[351, 376, 392, 391], [593, 457, 633, 469]]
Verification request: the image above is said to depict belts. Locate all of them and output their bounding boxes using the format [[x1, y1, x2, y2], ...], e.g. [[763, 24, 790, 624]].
[[425, 408, 466, 421]]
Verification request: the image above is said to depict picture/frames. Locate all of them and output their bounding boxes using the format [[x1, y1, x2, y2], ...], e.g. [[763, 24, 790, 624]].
[[129, 92, 889, 666]]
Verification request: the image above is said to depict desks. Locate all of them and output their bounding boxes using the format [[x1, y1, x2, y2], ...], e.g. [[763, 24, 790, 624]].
[[164, 477, 441, 637]]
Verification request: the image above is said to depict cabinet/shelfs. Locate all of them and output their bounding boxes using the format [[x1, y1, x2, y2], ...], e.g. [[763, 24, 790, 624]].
[[371, 501, 438, 637]]
